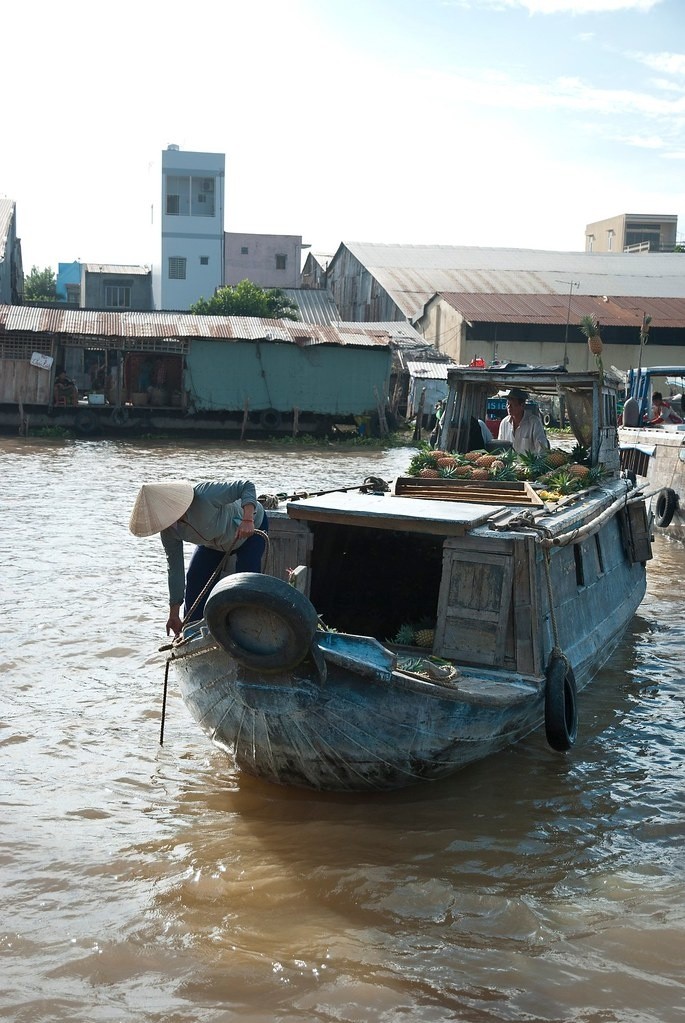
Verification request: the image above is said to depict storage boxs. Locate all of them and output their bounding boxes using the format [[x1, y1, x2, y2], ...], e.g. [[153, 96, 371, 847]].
[[471, 358, 485, 368], [88, 393, 104, 404]]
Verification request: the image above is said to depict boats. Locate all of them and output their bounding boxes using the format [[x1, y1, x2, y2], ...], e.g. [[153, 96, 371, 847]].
[[618, 366, 685, 542], [170, 357, 655, 790]]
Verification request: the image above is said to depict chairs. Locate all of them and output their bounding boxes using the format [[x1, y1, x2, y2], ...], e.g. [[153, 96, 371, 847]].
[[55, 385, 75, 407]]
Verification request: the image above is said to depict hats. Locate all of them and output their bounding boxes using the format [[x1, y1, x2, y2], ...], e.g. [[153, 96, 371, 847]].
[[129, 482, 194, 537], [501, 389, 528, 399]]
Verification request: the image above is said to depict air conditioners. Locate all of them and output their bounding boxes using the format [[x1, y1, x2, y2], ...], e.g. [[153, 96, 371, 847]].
[[201, 181, 214, 191]]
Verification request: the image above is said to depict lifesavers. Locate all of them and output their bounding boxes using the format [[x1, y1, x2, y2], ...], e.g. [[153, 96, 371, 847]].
[[202, 571, 318, 670], [111, 407, 129, 426], [259, 407, 281, 429], [544, 413, 553, 428], [654, 488, 676, 528], [72, 411, 97, 433], [545, 656, 578, 752]]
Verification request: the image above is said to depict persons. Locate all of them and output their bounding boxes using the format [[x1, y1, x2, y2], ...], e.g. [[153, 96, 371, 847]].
[[53, 371, 79, 407], [497, 388, 548, 459], [478, 419, 493, 443], [468, 416, 484, 451], [130, 480, 269, 636], [642, 392, 683, 425]]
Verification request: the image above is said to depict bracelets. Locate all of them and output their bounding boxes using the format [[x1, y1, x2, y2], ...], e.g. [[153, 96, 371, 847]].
[[243, 520, 254, 522]]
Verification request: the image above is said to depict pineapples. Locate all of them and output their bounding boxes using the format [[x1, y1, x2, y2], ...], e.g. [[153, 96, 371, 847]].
[[577, 314, 603, 354], [405, 443, 609, 494], [395, 617, 435, 648]]
